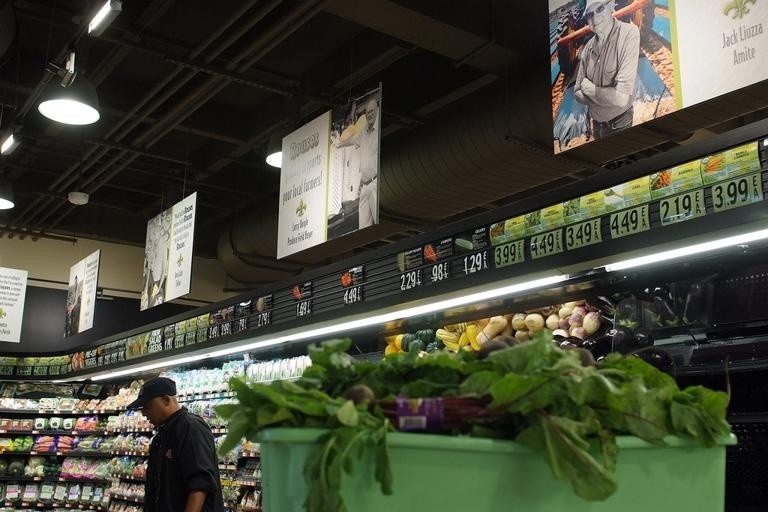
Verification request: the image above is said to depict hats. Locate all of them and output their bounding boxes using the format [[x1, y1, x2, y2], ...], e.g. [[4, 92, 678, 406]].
[[126, 377, 176, 410], [583, 0, 606, 16]]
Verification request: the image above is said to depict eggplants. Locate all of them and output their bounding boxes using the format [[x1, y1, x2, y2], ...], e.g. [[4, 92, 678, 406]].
[[552, 285, 672, 366]]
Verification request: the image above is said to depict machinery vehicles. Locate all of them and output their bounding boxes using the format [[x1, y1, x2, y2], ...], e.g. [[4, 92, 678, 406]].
[[556, 0, 654, 74]]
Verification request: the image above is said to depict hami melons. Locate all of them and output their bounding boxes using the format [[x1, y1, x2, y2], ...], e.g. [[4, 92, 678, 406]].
[[512, 313, 559, 342]]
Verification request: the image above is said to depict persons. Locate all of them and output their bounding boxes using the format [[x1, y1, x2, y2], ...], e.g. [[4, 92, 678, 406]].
[[333, 99, 378, 229], [573, 1, 641, 138], [126, 378, 224, 512], [68, 275, 82, 331], [144, 227, 167, 306]]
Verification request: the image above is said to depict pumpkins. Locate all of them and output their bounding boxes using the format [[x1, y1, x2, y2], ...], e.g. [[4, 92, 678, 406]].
[[385, 313, 513, 355]]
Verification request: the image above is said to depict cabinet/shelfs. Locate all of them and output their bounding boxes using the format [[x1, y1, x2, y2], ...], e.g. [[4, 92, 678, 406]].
[[0, 388, 261, 512]]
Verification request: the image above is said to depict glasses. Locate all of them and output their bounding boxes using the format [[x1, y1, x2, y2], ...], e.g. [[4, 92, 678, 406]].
[[586, 0, 612, 18]]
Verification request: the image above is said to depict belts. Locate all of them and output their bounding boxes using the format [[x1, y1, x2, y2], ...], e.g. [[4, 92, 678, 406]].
[[362, 176, 376, 185]]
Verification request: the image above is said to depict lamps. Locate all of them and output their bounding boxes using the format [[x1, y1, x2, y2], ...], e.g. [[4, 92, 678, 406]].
[[38, 74, 101, 125]]
